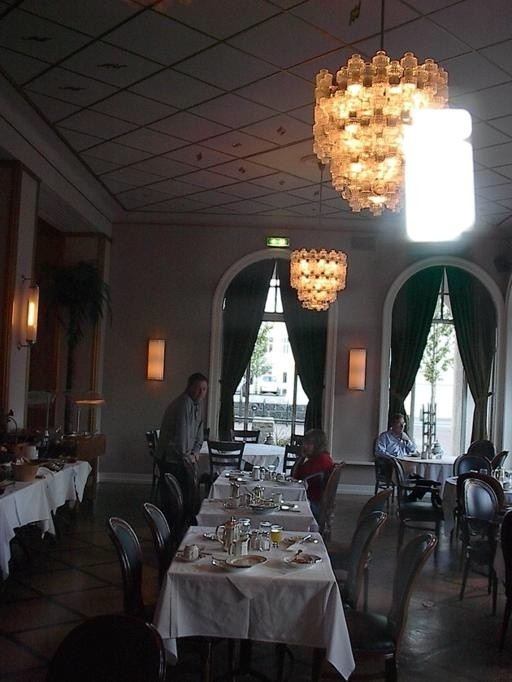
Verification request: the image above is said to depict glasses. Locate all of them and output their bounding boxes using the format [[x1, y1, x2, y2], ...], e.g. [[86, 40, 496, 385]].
[[397, 421, 406, 426]]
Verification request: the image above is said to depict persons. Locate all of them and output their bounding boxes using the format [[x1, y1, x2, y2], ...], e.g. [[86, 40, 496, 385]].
[[153, 372, 208, 539], [291, 428, 334, 520], [375, 412, 433, 504]]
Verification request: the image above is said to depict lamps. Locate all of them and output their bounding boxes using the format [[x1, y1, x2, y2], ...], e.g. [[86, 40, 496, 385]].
[[286, 155, 347, 314], [20, 275, 41, 351], [311, 0, 455, 220], [69, 388, 105, 435], [146, 338, 167, 382], [347, 348, 366, 391]]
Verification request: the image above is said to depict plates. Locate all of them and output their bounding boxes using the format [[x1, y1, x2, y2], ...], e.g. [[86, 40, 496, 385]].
[[176, 517, 323, 573]]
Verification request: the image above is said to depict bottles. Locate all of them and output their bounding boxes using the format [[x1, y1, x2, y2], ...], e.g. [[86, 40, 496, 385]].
[[225, 466, 284, 481], [44, 430, 49, 445], [479, 467, 512, 490], [420, 444, 440, 460]]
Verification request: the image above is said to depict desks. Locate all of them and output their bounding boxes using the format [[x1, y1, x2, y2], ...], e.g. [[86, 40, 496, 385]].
[[0, 458, 95, 581]]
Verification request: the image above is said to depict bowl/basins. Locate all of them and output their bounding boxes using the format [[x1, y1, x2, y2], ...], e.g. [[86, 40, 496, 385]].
[[11, 460, 39, 482]]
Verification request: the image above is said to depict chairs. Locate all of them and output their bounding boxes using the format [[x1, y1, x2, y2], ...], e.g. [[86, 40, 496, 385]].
[[54, 613, 170, 680], [106, 428, 511, 681]]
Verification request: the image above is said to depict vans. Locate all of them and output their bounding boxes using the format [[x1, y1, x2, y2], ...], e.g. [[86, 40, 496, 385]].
[[259, 374, 280, 396]]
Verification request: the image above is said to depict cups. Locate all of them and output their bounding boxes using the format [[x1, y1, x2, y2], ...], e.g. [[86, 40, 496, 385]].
[[222, 481, 302, 512]]
